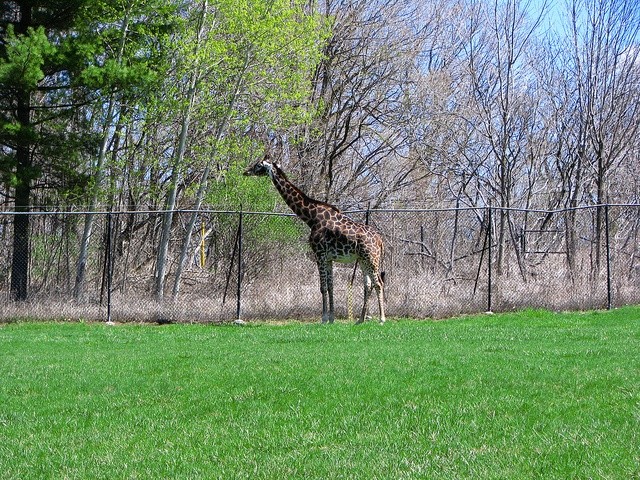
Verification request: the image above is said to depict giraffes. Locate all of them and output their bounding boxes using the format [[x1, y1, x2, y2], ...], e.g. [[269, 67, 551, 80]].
[[242, 154, 386, 326]]
